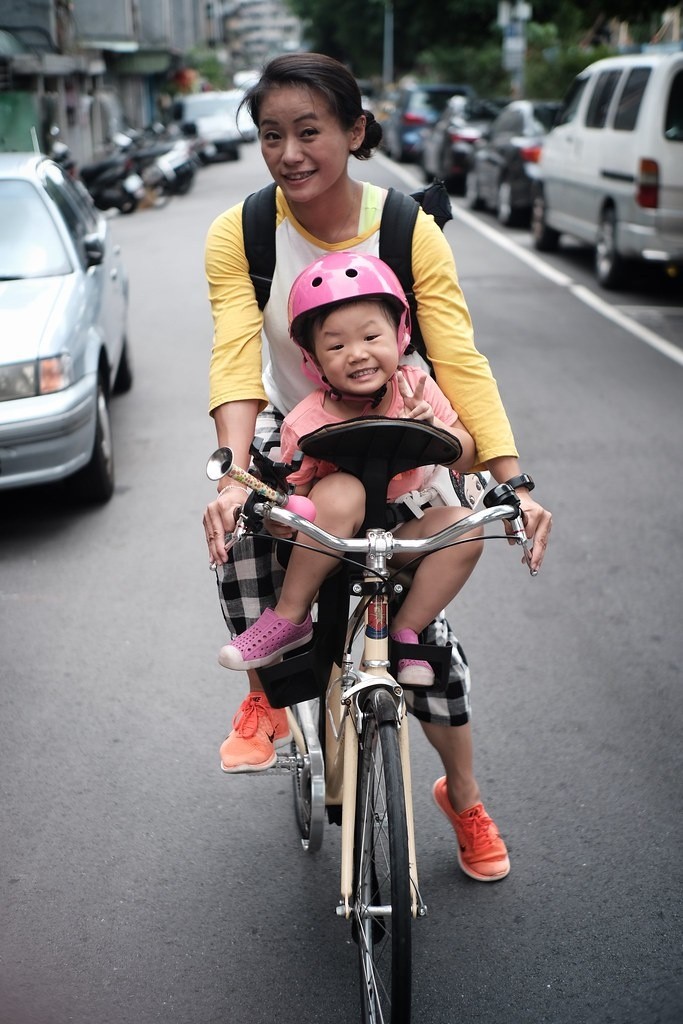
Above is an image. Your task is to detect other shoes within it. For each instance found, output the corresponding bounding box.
[218,608,314,671]
[389,628,434,686]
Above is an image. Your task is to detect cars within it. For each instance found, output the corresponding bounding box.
[0,151,135,505]
[465,98,562,225]
[421,94,511,193]
[356,79,476,160]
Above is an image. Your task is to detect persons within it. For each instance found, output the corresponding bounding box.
[203,55,552,880]
[218,252,484,686]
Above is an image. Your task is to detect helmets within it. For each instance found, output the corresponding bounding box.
[287,252,409,337]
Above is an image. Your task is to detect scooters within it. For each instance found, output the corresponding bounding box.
[47,120,208,215]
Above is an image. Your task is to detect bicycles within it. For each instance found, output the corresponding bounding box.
[208,490,538,1023]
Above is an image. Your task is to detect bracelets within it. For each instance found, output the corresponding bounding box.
[218,485,250,498]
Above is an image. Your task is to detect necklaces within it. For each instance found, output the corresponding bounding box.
[287,191,355,244]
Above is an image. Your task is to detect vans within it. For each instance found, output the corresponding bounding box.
[167,90,258,153]
[531,52,682,292]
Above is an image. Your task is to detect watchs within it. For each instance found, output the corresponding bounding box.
[505,474,535,492]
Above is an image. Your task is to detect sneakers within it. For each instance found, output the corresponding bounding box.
[219,692,292,773]
[432,775,510,882]
